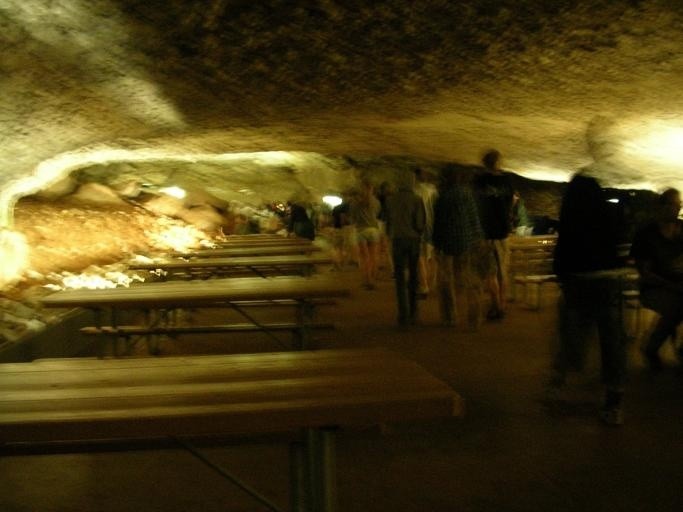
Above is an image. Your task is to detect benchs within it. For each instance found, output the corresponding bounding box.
[509,235,561,312]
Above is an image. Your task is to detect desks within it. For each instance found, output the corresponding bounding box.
[0,254,470,511]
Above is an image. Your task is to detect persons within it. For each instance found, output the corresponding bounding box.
[433,165,488,326]
[383,169,427,326]
[512,188,528,237]
[275,181,392,289]
[628,186,682,368]
[545,173,627,425]
[413,168,440,300]
[471,148,517,319]
